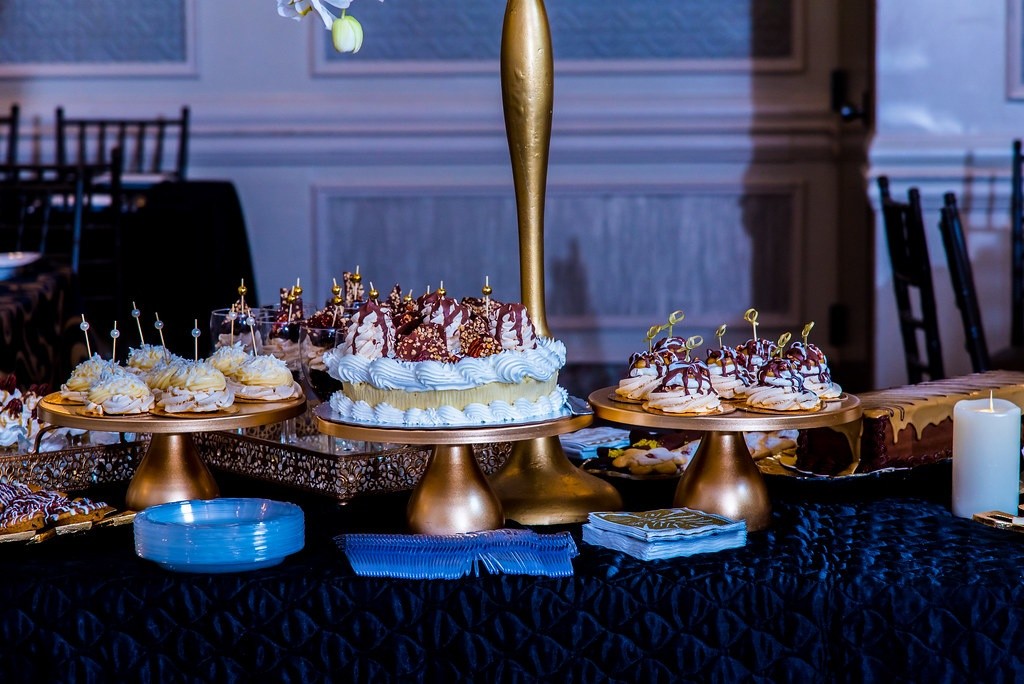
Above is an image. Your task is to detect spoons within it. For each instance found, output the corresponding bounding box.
[332,522,579,582]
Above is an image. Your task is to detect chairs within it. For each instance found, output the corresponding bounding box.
[52,104,193,181]
[0,142,143,362]
[873,137,1023,385]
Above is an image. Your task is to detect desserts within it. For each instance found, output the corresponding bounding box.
[0,273,1024,542]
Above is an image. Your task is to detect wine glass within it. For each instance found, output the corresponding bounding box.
[211,297,391,456]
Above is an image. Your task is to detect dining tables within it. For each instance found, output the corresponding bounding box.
[0,356,1023,682]
[0,173,263,396]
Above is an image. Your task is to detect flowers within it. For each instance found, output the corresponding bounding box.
[277,0,364,56]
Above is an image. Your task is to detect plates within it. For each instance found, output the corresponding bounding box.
[130,495,306,577]
